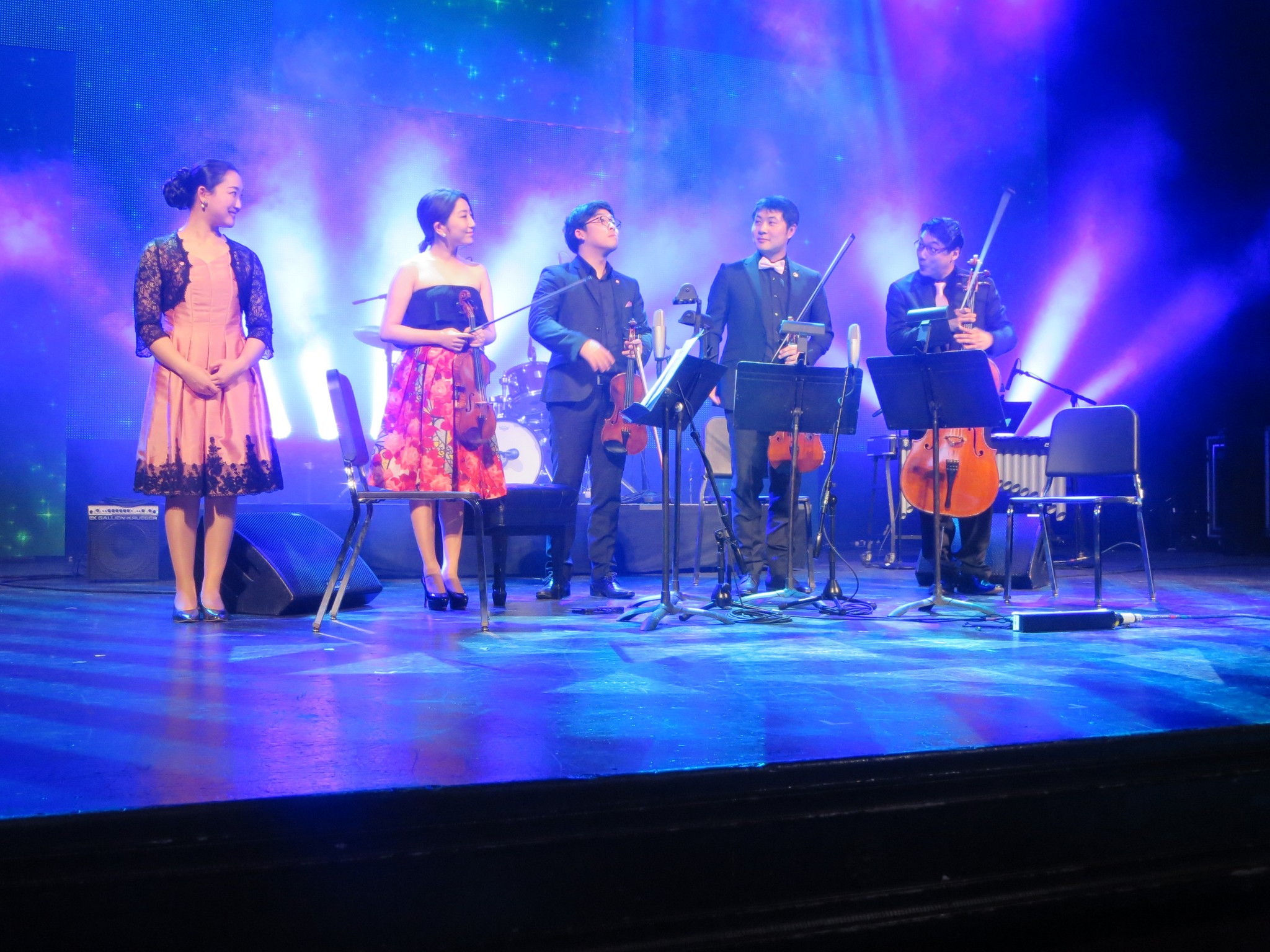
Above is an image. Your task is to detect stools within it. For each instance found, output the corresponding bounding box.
[431,479,568,606]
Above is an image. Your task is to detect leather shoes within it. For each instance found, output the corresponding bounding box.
[928,580,955,595]
[589,571,635,598]
[957,574,1004,594]
[536,571,570,599]
[765,578,813,594]
[736,573,758,595]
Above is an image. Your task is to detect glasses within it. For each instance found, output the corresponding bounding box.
[914,240,945,255]
[585,216,621,229]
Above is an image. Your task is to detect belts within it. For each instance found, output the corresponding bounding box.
[595,374,609,385]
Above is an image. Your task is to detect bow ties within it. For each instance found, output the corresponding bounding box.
[758,256,785,275]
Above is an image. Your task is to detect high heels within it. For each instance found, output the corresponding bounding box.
[444,583,469,609]
[421,576,449,611]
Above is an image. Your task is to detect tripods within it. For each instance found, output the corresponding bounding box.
[617,348,1015,631]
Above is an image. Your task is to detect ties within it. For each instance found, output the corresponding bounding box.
[934,283,948,307]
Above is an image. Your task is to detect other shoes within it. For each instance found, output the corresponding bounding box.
[172,603,200,623]
[199,590,229,622]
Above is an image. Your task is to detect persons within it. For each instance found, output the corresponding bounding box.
[885,217,1019,596]
[701,195,835,597]
[131,158,286,622]
[364,187,507,611]
[527,200,654,598]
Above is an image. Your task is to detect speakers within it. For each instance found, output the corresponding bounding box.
[914,514,1054,589]
[194,513,384,617]
[89,505,161,582]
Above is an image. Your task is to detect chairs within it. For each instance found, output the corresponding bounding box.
[693,416,815,592]
[311,368,489,634]
[1003,405,1160,607]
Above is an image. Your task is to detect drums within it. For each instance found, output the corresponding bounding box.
[494,418,544,484]
[505,361,549,415]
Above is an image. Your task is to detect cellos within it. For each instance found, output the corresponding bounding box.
[899,254,1002,557]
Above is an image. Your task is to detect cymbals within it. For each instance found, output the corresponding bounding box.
[489,359,497,372]
[353,324,403,352]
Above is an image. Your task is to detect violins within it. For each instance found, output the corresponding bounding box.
[601,318,648,456]
[767,317,826,475]
[453,289,497,448]
